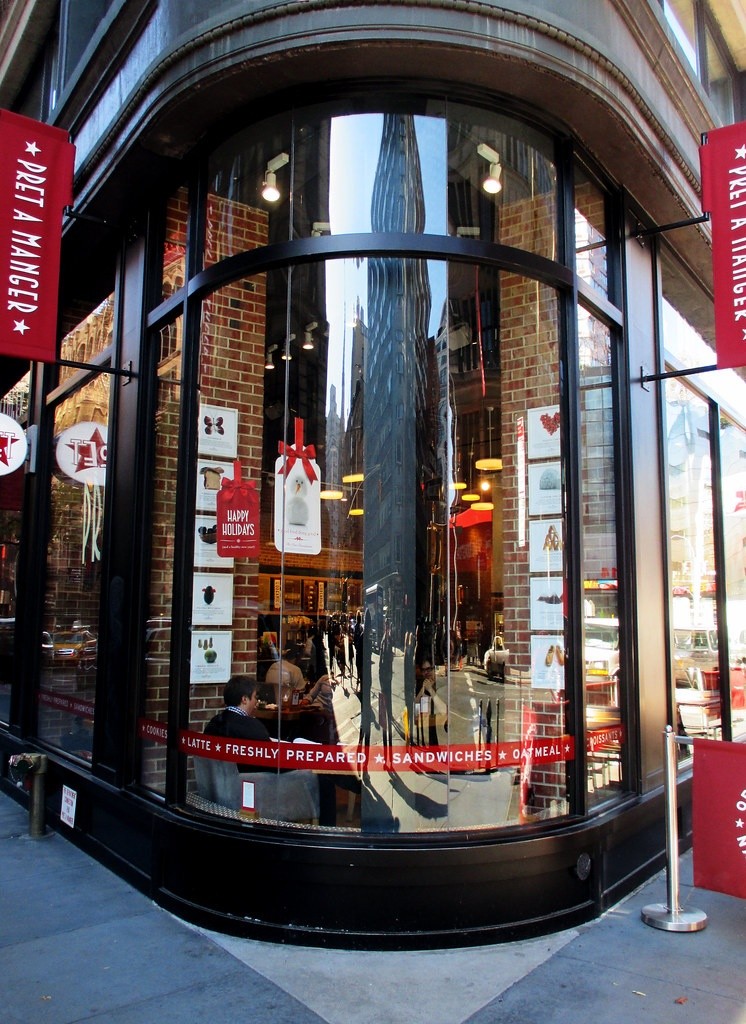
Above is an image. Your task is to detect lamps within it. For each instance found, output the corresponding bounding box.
[320,400,503,517]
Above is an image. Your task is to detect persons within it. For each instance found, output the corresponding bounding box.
[204,673,299,775]
[312,606,503,771]
[299,657,358,745]
[266,645,307,701]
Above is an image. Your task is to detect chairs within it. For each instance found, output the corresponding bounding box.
[194,753,319,825]
